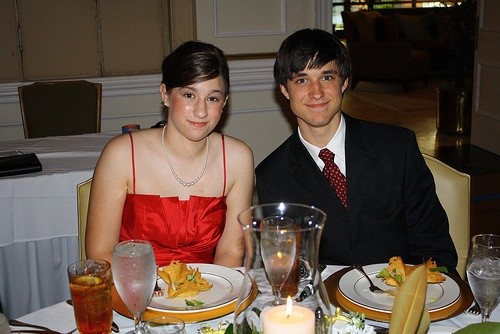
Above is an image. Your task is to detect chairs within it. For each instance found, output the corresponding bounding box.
[422,152,471,280]
[18,79,102,138]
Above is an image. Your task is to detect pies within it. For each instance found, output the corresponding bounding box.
[157,259,213,299]
[376,255,446,292]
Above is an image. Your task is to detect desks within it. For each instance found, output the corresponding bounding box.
[0,131,130,320]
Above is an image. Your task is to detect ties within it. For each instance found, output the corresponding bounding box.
[318,148,347,206]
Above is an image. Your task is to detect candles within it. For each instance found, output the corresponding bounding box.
[261,294,316,334]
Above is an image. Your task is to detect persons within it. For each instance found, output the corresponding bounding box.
[86,40,255,284]
[251,29,467,290]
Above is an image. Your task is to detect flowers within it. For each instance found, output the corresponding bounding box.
[201,306,376,334]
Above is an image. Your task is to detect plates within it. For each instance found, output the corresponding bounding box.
[337,262,462,314]
[145,263,252,314]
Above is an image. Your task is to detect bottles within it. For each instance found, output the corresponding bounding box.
[232,203,332,334]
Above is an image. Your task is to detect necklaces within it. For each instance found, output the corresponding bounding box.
[162,124,209,186]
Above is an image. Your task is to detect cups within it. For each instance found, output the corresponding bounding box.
[144,316,186,334]
[67,259,114,334]
[122,124,140,134]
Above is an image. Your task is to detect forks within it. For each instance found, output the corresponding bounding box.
[467,303,481,315]
[353,263,386,295]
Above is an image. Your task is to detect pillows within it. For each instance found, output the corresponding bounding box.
[356,8,441,43]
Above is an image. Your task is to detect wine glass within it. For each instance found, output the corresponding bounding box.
[466,234,500,322]
[259,216,296,308]
[111,239,157,334]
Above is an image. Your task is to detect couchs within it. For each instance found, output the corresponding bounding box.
[343,9,464,91]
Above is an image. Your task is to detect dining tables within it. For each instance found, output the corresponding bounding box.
[7,266,500,334]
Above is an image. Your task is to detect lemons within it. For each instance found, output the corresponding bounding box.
[71,275,102,291]
